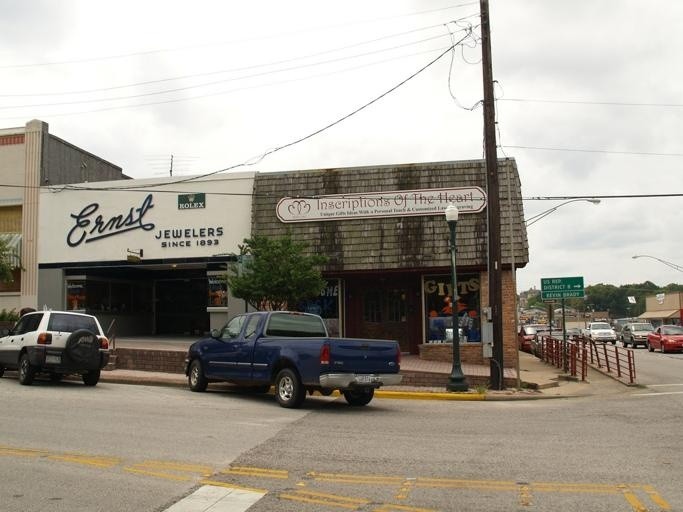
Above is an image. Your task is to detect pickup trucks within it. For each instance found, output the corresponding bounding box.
[178,309,406,409]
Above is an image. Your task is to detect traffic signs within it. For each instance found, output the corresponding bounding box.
[540,276,586,300]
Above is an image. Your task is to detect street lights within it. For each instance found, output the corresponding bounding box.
[522,198,605,226]
[631,254,683,278]
[438,204,471,392]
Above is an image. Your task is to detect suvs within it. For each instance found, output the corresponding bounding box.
[0,304,111,392]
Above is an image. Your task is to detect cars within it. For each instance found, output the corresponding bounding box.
[516,321,683,358]
